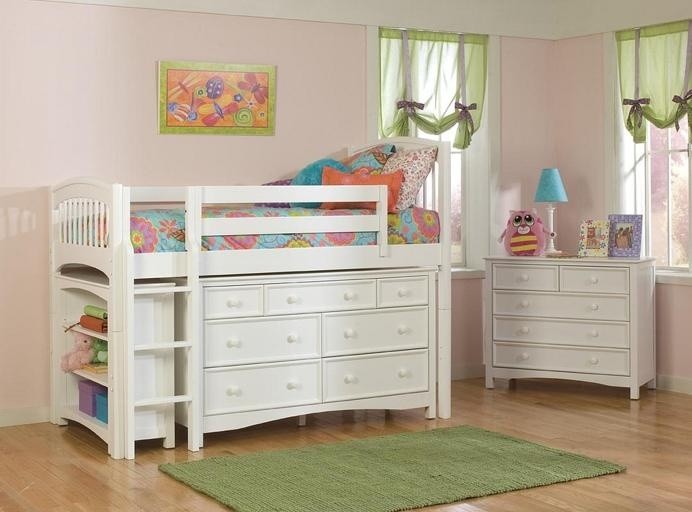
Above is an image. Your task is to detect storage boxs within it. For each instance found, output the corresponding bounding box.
[77,379,109,424]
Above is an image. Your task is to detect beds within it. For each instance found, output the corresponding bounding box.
[51,135,450,276]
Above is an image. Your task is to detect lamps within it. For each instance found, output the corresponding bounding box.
[535,167,567,257]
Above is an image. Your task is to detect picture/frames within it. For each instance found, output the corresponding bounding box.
[578,216,640,256]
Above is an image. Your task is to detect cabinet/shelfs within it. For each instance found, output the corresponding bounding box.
[177,267,451,452]
[47,271,175,460]
[484,253,656,399]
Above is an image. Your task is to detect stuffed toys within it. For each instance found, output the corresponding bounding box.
[61,333,95,373]
[497,208,557,256]
[89,338,108,365]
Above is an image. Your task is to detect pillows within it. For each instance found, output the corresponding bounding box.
[249,142,437,212]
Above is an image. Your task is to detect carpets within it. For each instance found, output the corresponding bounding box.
[160,423,627,511]
